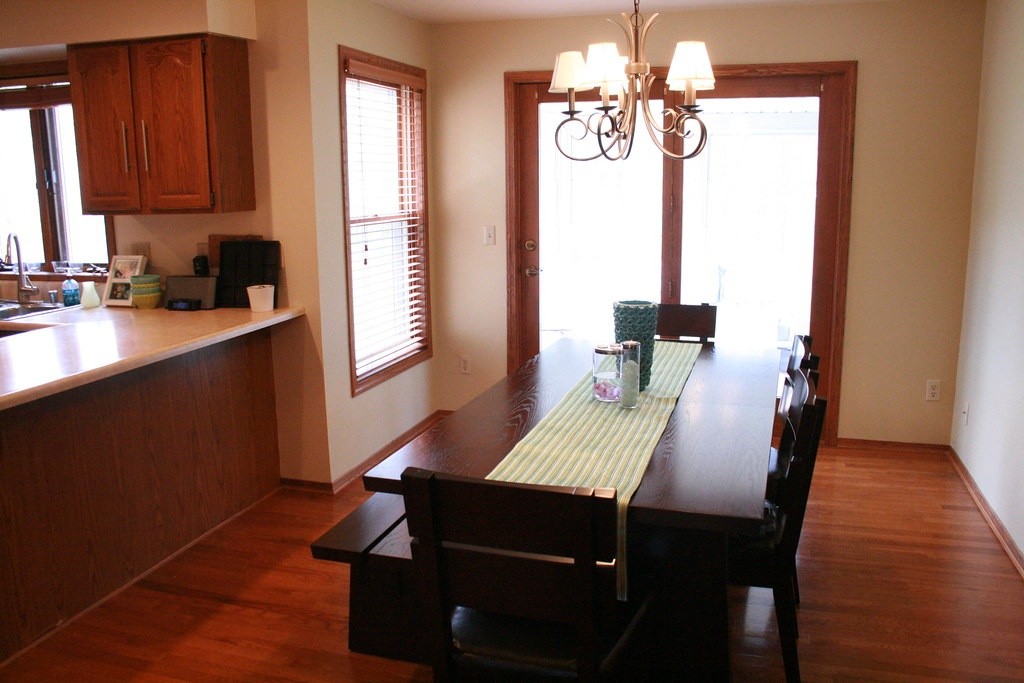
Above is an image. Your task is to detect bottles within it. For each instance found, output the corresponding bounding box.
[80,281,100,309]
[62,275,80,307]
[620,340,640,409]
[593,344,621,403]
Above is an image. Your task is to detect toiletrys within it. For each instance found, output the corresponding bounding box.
[61,267,80,306]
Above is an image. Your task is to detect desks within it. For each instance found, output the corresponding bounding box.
[364,337,782,683]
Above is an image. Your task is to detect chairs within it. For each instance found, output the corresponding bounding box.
[400,306,826,683]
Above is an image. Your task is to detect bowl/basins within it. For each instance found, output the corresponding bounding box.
[130,274,162,310]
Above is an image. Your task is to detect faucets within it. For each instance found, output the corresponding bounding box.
[5,233,39,303]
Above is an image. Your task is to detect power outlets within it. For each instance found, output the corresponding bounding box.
[926,380,941,401]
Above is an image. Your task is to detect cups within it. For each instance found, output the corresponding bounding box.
[51,260,83,274]
[247,285,275,312]
[12,257,41,272]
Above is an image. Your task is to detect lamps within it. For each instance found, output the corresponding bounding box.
[549,0,717,161]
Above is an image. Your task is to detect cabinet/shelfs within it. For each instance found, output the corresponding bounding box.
[67,33,257,216]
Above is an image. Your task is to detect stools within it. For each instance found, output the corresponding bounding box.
[310,490,452,663]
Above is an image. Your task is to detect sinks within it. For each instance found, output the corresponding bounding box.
[0,301,83,322]
